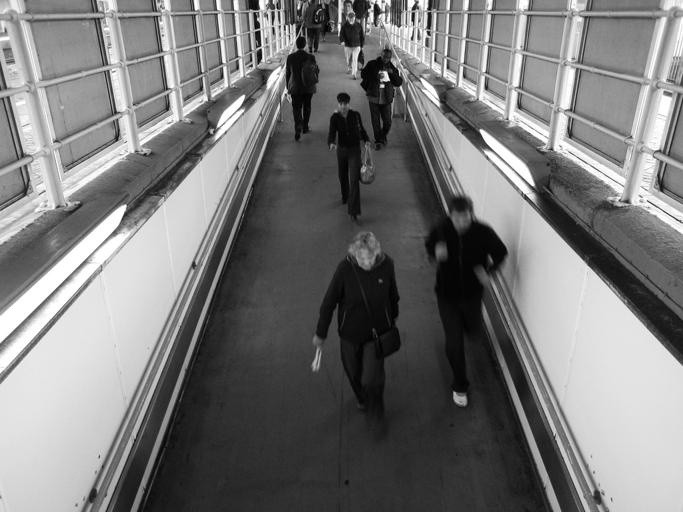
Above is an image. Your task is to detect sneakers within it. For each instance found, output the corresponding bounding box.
[357,398,369,411]
[375,135,388,151]
[453,390,468,408]
[348,68,357,81]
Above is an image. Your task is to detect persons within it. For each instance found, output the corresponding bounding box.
[286,37,316,141]
[374,0,382,27]
[361,48,403,151]
[312,232,400,436]
[384,2,390,24]
[410,0,422,41]
[339,12,365,80]
[327,93,372,222]
[425,196,509,408]
[263,0,372,54]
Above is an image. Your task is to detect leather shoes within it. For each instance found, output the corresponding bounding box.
[351,214,357,224]
[295,127,311,140]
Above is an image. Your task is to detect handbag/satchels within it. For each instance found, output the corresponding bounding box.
[374,327,401,359]
[312,4,325,24]
[360,159,376,184]
[358,51,365,66]
[301,63,319,88]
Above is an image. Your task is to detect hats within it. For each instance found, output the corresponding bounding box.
[337,93,351,102]
